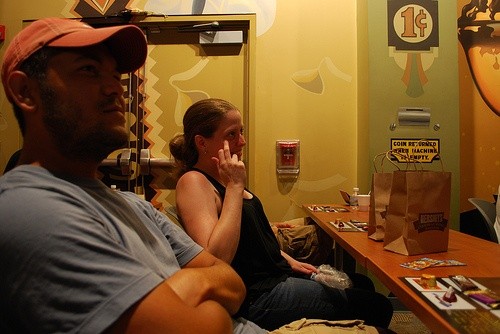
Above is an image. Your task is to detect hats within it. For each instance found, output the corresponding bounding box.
[0,17,148,102]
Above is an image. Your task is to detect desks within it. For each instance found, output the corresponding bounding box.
[302,205,500,334]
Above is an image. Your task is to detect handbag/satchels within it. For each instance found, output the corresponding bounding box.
[367,139,453,256]
[277,224,334,266]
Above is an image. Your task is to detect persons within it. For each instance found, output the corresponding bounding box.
[169,98,394,329]
[0,18,396,334]
